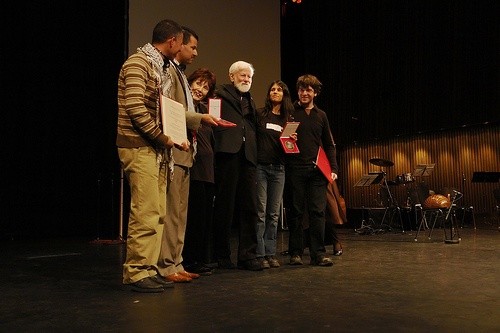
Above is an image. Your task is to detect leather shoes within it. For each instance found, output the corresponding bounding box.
[125,277,165,293]
[150,275,175,287]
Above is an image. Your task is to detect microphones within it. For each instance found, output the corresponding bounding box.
[452,189,462,194]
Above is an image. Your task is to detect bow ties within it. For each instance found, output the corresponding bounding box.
[178,64,187,71]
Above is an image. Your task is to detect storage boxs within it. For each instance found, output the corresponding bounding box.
[280,122,300,154]
[208,97,237,127]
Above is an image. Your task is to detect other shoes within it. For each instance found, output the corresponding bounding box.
[187,265,211,275]
[333,245,343,256]
[310,256,333,266]
[268,258,280,267]
[178,271,200,278]
[238,258,269,270]
[164,272,192,282]
[290,255,303,265]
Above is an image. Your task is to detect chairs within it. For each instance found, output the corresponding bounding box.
[414,189,463,241]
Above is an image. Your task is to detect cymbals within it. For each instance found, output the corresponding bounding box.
[369,159,394,167]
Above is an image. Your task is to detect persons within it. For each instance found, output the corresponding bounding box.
[156,25,221,283]
[116,19,191,292]
[214,60,264,270]
[287,74,337,266]
[186,66,216,274]
[280,181,343,256]
[255,80,298,268]
